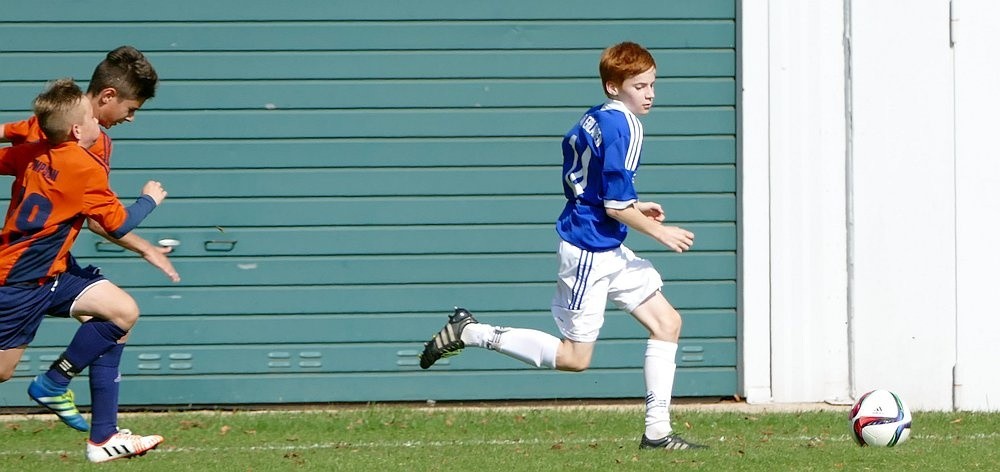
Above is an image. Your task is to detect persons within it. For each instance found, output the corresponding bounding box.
[417,42,712,452]
[0,77,165,432]
[0,45,182,464]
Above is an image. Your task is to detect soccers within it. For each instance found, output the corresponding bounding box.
[849,388,913,447]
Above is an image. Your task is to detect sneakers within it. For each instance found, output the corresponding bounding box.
[86,426,164,463]
[27,374,89,432]
[639,430,710,452]
[419,307,478,369]
